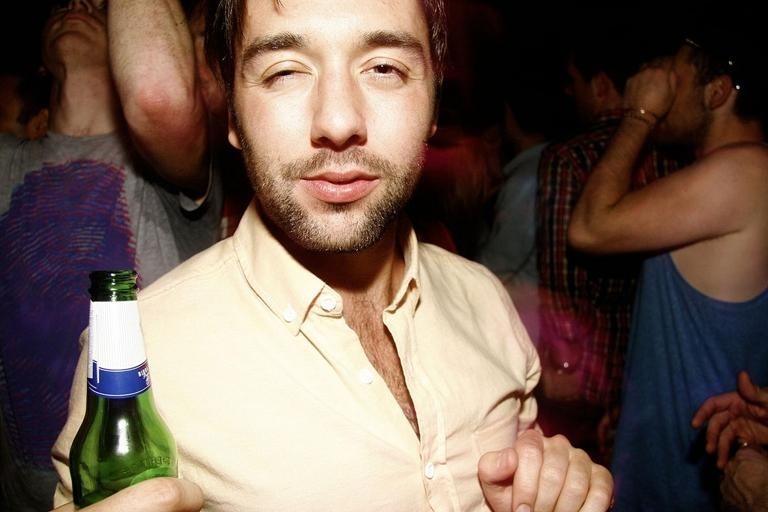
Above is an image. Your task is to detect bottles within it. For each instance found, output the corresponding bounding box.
[68,268,186,507]
